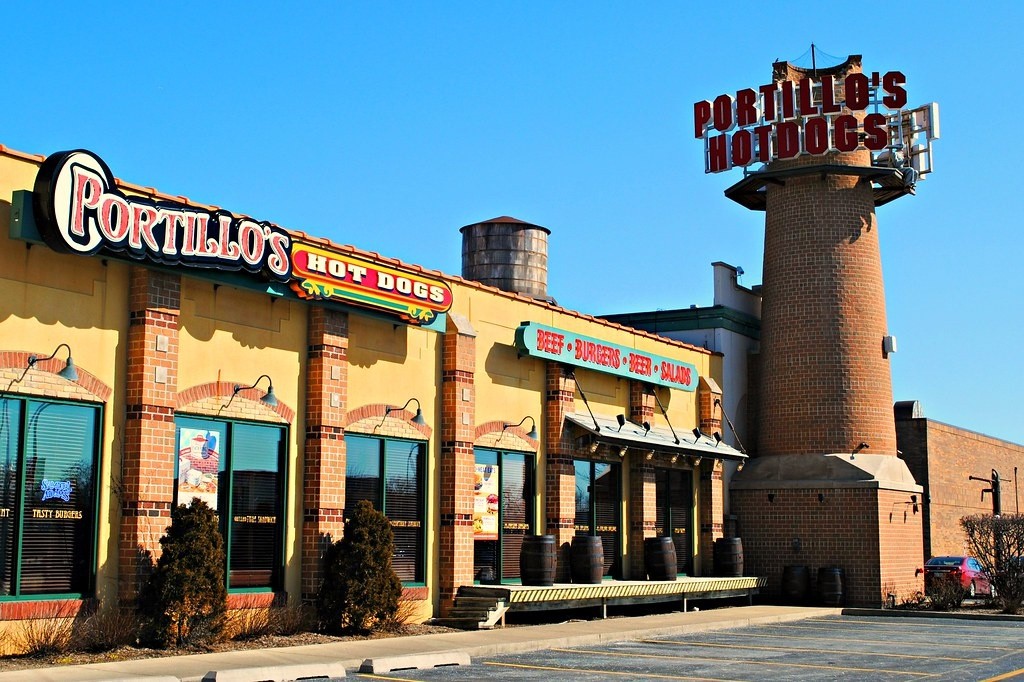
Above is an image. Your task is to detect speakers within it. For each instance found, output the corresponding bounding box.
[885,335,896,353]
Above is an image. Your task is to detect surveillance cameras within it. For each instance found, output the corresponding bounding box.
[737,266,744,274]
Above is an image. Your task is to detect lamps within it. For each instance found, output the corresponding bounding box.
[713,431,722,447]
[737,457,745,472]
[645,448,656,460]
[897,449,902,455]
[906,495,919,505]
[234,374,279,407]
[670,451,679,464]
[386,397,425,425]
[28,344,79,381]
[643,421,650,437]
[589,436,601,453]
[716,457,724,468]
[768,493,776,503]
[503,415,539,440]
[618,444,628,458]
[818,493,825,503]
[860,441,869,449]
[616,414,625,433]
[692,427,701,444]
[693,454,703,466]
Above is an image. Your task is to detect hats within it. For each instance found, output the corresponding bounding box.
[894,139,904,145]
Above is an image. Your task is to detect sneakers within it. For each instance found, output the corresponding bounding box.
[904,186,916,196]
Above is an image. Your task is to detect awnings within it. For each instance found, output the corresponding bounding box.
[564,413,750,462]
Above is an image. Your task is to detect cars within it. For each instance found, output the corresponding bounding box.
[925,556,998,599]
[988,556,1024,592]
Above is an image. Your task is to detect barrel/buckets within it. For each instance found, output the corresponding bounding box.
[817,567,845,607]
[644,536,678,580]
[715,536,744,577]
[782,567,811,605]
[571,535,604,584]
[520,534,557,587]
